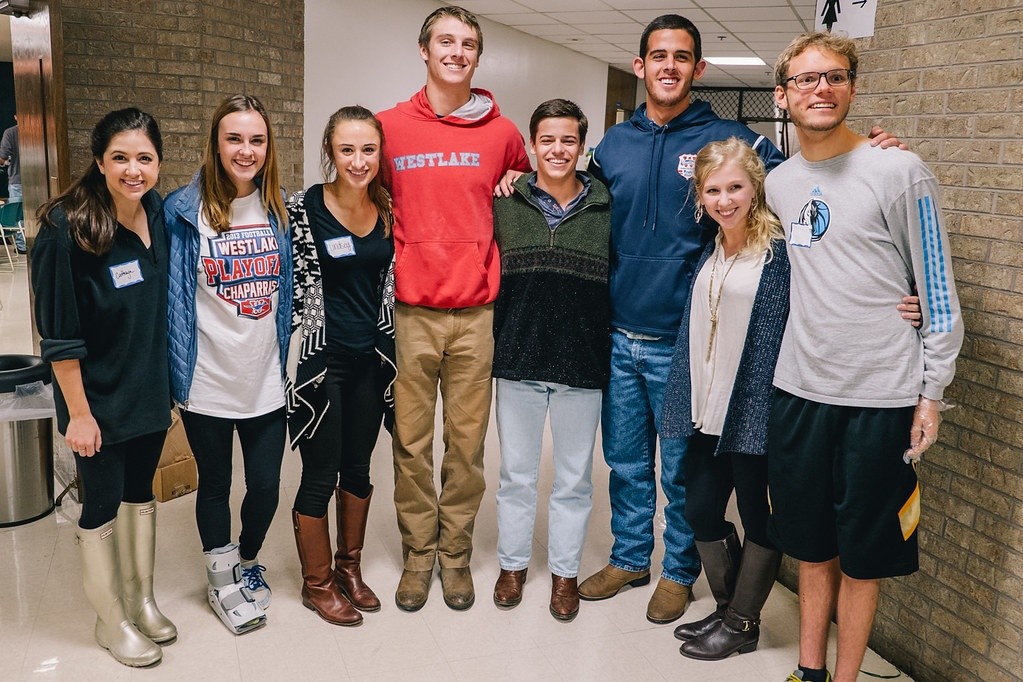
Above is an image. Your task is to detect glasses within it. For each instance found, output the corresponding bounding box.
[786,69,854,90]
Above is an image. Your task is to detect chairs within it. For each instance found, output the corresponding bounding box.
[0,201,26,273]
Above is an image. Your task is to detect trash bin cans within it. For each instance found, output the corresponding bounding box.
[0,354,55,527]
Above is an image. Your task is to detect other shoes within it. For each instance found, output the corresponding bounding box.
[784,669,832,682]
[646,577,692,624]
[577,565,650,601]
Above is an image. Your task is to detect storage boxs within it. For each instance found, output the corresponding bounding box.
[156,404,195,469]
[153,456,198,503]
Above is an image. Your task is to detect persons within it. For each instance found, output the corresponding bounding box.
[164,93,293,637]
[654,137,921,663]
[491,97,613,622]
[754,32,966,682]
[0,111,27,254]
[371,4,534,613]
[29,106,180,669]
[284,105,399,627]
[493,13,910,624]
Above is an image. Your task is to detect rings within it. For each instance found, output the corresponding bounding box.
[78,449,85,451]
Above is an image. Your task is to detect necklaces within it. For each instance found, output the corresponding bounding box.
[704,223,743,365]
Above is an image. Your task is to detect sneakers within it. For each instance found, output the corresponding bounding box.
[241,558,271,609]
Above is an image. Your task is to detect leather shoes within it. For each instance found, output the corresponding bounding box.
[494,567,528,605]
[395,569,432,611]
[550,573,579,620]
[440,567,475,610]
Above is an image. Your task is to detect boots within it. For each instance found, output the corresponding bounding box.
[674,521,742,641]
[292,507,363,626]
[116,495,178,642]
[680,536,782,661]
[76,518,163,667]
[334,485,381,612]
